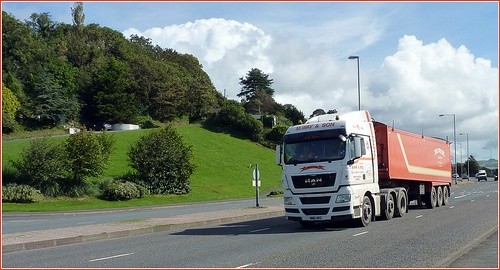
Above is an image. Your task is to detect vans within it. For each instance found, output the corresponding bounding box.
[477,170,488,181]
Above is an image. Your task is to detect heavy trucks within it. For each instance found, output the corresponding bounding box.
[277,110,452,227]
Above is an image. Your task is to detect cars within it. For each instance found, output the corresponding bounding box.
[475,173,478,178]
[452,174,459,178]
[462,174,469,179]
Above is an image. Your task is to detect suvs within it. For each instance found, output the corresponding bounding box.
[493,169,498,181]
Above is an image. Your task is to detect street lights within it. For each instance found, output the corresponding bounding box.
[482,147,492,159]
[348,56,360,111]
[438,114,458,184]
[451,142,462,163]
[459,133,469,180]
